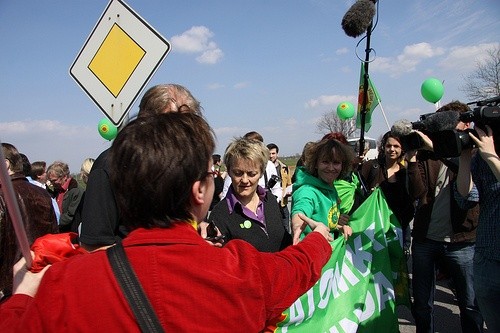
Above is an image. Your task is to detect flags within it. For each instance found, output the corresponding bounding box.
[355,62,382,132]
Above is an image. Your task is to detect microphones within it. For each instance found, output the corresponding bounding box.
[423,111,473,130]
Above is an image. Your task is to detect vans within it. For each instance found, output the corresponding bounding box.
[346,137,380,162]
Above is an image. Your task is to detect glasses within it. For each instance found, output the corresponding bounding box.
[208,165,221,179]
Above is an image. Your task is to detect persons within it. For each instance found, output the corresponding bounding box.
[356,102,500,333]
[291,138,355,245]
[220,131,282,203]
[80,84,223,249]
[0,112,331,333]
[324,132,364,223]
[267,143,291,207]
[0,144,100,300]
[209,137,289,252]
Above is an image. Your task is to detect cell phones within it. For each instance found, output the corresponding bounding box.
[206,235,225,240]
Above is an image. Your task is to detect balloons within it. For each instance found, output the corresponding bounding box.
[337,102,354,120]
[98,117,117,142]
[421,78,445,104]
